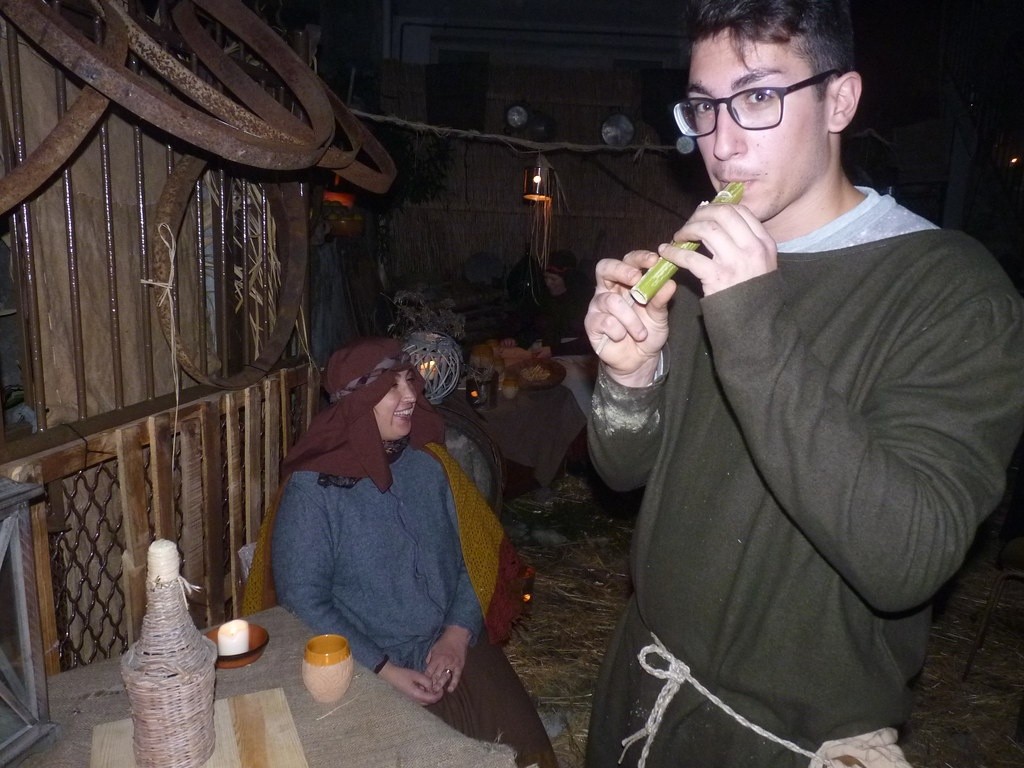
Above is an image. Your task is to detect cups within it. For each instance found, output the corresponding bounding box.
[495,359,504,390]
[467,369,498,412]
[302,634,351,702]
[471,346,494,368]
[503,379,519,399]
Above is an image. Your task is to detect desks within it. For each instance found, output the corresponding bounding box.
[0,607,519,768]
[448,354,591,489]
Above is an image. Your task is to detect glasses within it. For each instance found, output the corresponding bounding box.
[674,69,842,138]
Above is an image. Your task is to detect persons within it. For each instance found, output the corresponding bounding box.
[493,251,594,354]
[241,339,560,768]
[581,0,1024,768]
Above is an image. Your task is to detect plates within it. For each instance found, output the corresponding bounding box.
[510,359,566,392]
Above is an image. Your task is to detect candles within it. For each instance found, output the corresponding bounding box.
[218,619,249,656]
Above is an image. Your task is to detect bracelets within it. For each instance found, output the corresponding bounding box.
[375,654,389,673]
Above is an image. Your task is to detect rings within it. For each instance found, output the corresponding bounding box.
[443,669,452,673]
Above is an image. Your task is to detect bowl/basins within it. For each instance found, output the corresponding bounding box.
[203,623,270,668]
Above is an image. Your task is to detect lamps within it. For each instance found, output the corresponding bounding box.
[522,151,551,202]
[402,331,460,407]
[465,367,499,411]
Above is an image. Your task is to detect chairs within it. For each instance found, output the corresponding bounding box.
[239,442,528,646]
[961,537,1024,683]
[439,404,507,520]
